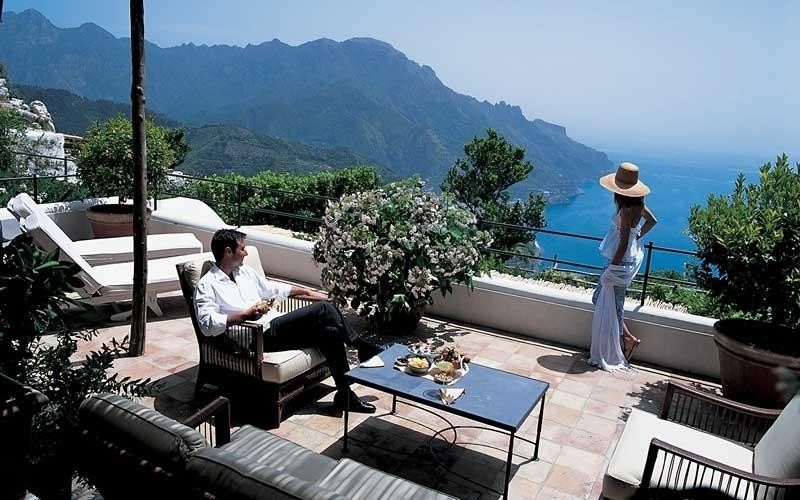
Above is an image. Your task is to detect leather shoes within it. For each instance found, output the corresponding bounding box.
[357,341,396,364]
[332,389,377,414]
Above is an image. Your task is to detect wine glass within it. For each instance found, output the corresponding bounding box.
[436,361,454,385]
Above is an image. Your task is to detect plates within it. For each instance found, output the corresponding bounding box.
[408,342,436,357]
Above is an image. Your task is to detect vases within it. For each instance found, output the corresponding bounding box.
[376,300,427,334]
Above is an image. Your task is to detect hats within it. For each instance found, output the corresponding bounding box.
[597,161,651,198]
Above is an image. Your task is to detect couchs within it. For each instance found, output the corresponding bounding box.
[601,375,799,498]
[75,391,459,500]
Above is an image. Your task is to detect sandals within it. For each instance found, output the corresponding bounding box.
[586,358,604,371]
[623,335,642,361]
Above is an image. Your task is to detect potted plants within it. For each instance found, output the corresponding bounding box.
[683,154,800,409]
[74,115,178,238]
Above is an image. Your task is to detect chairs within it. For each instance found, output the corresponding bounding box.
[6,193,203,266]
[17,213,266,322]
[177,245,350,429]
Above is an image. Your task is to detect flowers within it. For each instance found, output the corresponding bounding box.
[312,174,494,318]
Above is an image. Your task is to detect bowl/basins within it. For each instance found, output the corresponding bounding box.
[407,364,430,374]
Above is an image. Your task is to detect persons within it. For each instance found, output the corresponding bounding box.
[584,191,657,373]
[193,230,402,413]
